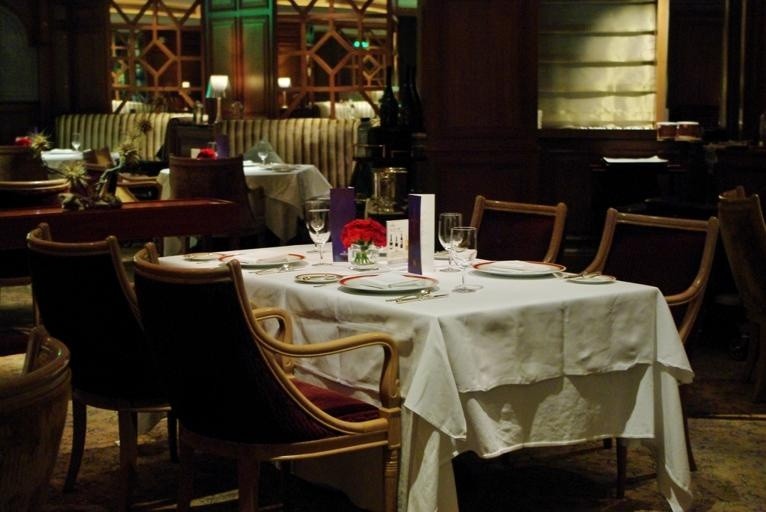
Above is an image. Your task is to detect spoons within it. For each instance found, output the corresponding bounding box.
[386,288,431,301]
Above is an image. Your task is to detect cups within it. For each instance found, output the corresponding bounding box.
[206,140,218,153]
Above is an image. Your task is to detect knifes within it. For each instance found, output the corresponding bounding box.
[392,294,449,304]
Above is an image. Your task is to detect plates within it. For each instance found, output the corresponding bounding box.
[296,272,341,284]
[219,252,304,266]
[567,274,617,286]
[341,273,438,294]
[184,252,225,261]
[470,260,567,278]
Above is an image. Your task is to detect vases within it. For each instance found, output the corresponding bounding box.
[347,244,376,267]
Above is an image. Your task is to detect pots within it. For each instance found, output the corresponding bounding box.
[369,165,413,211]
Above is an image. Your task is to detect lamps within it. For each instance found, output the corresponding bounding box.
[275,76,291,110]
[659,121,705,163]
[207,74,233,124]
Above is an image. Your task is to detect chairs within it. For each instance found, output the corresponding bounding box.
[0,132,169,205]
[169,154,254,230]
[0,328,70,512]
[578,206,719,499]
[719,185,766,348]
[131,243,402,511]
[16,223,179,488]
[462,194,568,265]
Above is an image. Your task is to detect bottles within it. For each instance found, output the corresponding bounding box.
[192,102,203,124]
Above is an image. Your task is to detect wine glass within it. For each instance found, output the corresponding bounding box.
[70,132,81,153]
[306,208,337,267]
[448,225,480,294]
[256,141,269,167]
[302,198,332,253]
[438,210,464,272]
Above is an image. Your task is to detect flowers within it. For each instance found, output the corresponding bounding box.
[193,149,220,159]
[339,219,386,262]
